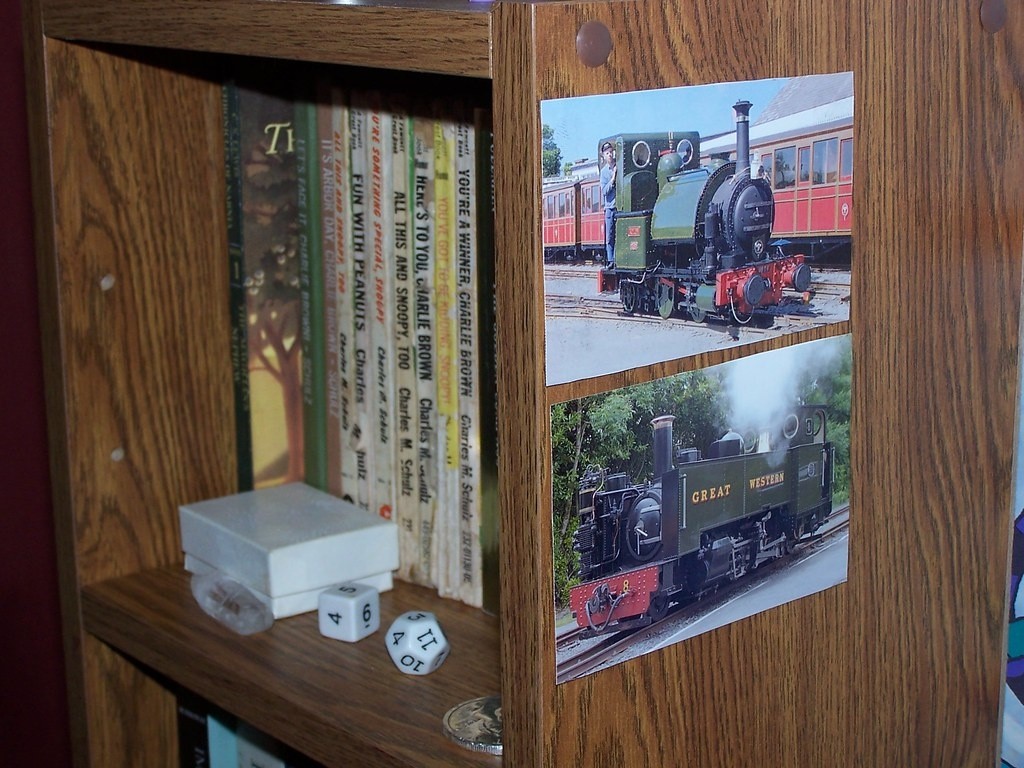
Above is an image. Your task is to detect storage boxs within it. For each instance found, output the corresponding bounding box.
[179,484,401,621]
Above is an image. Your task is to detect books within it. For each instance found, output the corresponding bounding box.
[218,73,499,609]
[175,699,285,768]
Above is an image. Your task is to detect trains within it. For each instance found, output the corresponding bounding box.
[542,115,853,264]
[568,403,835,639]
[598,98,815,327]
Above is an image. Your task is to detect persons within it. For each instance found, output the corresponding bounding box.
[596,142,618,269]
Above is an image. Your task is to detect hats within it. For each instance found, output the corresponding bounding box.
[602,142,611,152]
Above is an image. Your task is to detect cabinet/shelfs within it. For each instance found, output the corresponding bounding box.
[20,0,1024,768]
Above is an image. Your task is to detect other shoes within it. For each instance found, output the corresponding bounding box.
[608,262,614,268]
